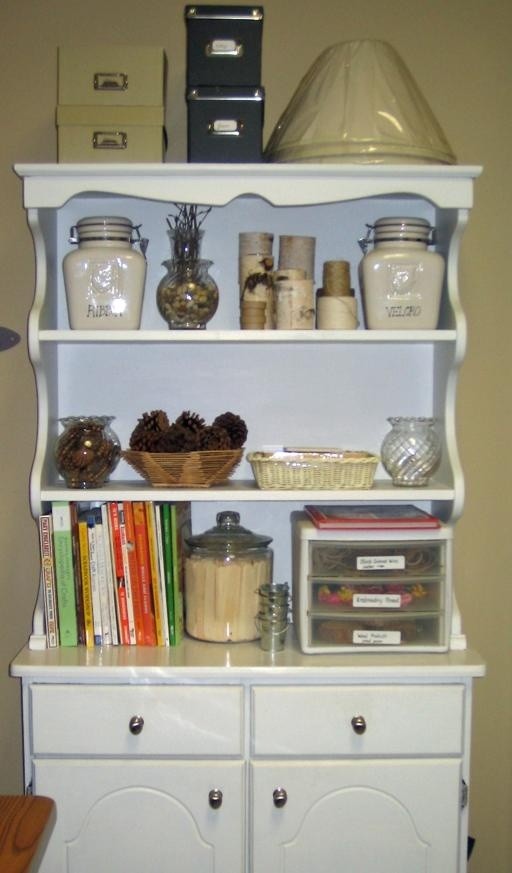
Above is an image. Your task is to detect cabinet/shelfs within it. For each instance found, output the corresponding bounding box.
[11,153,482,653]
[4,621,488,873]
[290,509,455,654]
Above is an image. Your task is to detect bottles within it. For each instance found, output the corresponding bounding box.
[357,216,445,330]
[53,415,121,489]
[380,416,442,487]
[184,509,274,644]
[61,215,147,330]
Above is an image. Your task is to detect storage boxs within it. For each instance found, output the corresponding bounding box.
[183,3,266,165]
[53,40,169,163]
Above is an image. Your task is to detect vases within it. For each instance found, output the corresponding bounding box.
[156,228,219,329]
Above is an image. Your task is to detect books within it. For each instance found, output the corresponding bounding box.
[304,502,443,532]
[38,494,193,650]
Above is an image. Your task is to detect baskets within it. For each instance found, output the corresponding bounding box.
[121,449,245,489]
[246,449,379,492]
